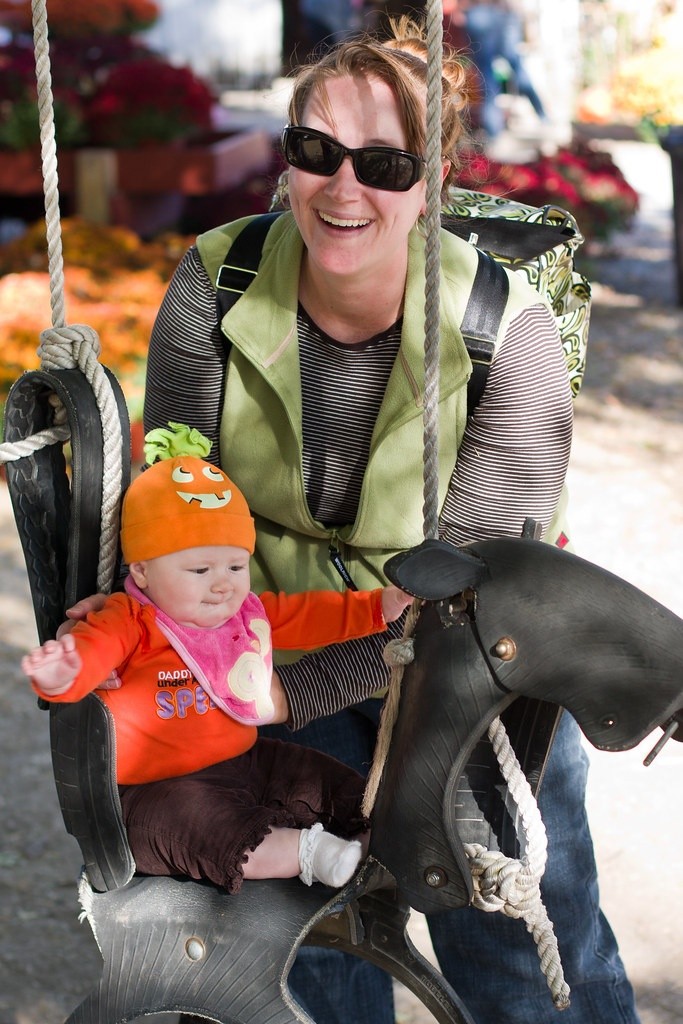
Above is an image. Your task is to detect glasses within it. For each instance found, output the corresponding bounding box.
[281,122,428,192]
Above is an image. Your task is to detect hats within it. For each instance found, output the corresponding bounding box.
[119,421,256,565]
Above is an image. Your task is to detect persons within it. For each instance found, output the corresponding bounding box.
[19,422,427,894]
[56,15,642,1024]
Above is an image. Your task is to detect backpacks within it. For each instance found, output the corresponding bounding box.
[214,167,591,401]
